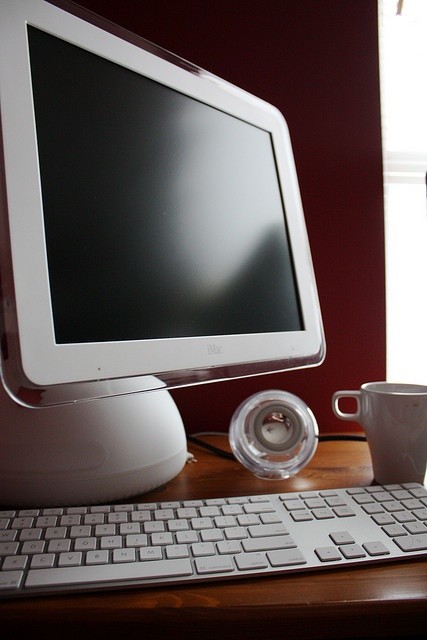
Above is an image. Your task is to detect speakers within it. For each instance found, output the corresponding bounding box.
[228,388,321,481]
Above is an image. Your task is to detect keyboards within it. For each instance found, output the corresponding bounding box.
[0,481,427,600]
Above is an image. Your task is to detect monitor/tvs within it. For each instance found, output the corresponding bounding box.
[0,0,328,508]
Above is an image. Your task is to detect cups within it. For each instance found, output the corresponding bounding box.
[332,381,427,485]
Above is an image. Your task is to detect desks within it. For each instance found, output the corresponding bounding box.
[1,432,426,640]
